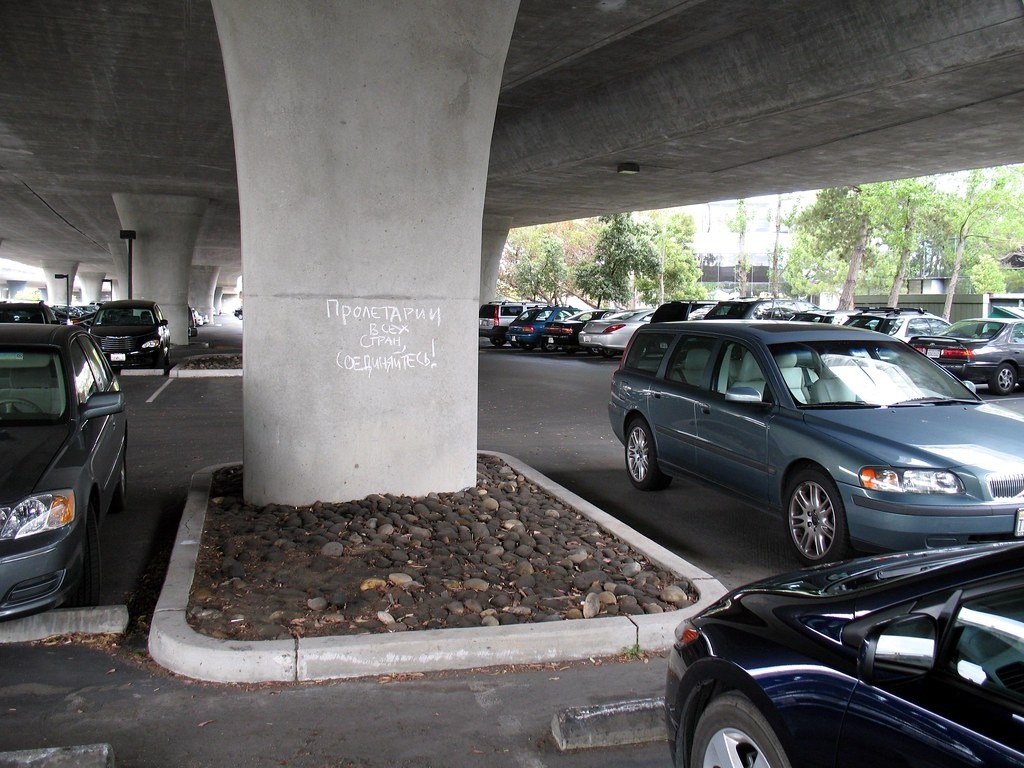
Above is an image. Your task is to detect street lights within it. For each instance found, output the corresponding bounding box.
[55,274,70,318]
[120,229,137,299]
[101,279,112,302]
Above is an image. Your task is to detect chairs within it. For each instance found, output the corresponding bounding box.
[811,358,859,403]
[765,352,810,406]
[6,368,53,412]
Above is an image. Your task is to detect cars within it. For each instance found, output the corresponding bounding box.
[505,305,589,351]
[907,317,1024,396]
[608,319,1024,567]
[577,308,657,358]
[0,322,127,623]
[0,300,208,339]
[540,309,621,355]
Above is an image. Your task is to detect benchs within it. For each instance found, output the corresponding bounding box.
[670,350,766,394]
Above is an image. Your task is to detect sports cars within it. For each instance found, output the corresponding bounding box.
[663,535,1024,768]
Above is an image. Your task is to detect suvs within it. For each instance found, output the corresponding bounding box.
[653,296,952,361]
[84,299,170,373]
[479,300,573,348]
